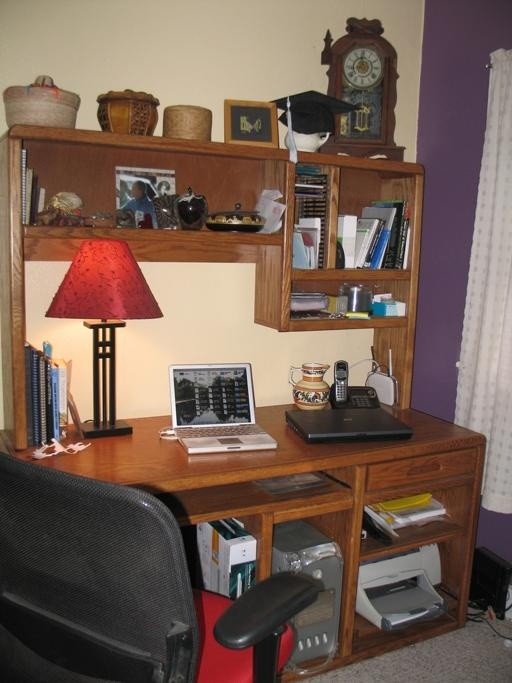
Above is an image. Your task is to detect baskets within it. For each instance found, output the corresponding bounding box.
[4,72,82,132]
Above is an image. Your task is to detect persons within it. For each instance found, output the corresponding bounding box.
[152,181,177,229]
[120,181,158,228]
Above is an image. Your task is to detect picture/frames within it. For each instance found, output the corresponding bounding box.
[221,99,282,151]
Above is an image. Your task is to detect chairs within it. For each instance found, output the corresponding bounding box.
[1,449,319,683]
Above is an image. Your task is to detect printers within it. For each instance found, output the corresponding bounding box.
[355,543,448,632]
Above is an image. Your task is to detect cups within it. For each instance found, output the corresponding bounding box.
[342,286,369,313]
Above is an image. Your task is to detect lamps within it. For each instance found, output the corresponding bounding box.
[45,241,169,439]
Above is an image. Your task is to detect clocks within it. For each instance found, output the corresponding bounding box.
[320,15,406,161]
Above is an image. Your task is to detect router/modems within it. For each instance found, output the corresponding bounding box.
[367,345,399,406]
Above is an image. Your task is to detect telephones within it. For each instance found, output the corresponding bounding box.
[329,360,380,409]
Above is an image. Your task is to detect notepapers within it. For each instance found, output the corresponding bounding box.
[346,312,371,320]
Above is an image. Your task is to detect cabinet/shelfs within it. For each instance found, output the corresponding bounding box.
[1,125,489,683]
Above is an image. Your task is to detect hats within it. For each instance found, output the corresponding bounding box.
[268,89,359,133]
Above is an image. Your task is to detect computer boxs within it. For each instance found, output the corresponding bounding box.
[271,521,343,669]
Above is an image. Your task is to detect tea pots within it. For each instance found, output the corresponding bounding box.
[287,361,332,411]
[284,128,330,153]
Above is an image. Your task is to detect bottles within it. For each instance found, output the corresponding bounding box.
[174,187,208,231]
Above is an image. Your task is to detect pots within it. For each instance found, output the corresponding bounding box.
[204,204,265,233]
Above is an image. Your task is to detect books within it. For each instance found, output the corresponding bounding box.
[369,493,447,531]
[292,165,410,271]
[20,149,46,227]
[24,339,71,448]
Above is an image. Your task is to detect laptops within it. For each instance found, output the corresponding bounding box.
[285,407,413,444]
[170,363,278,454]
